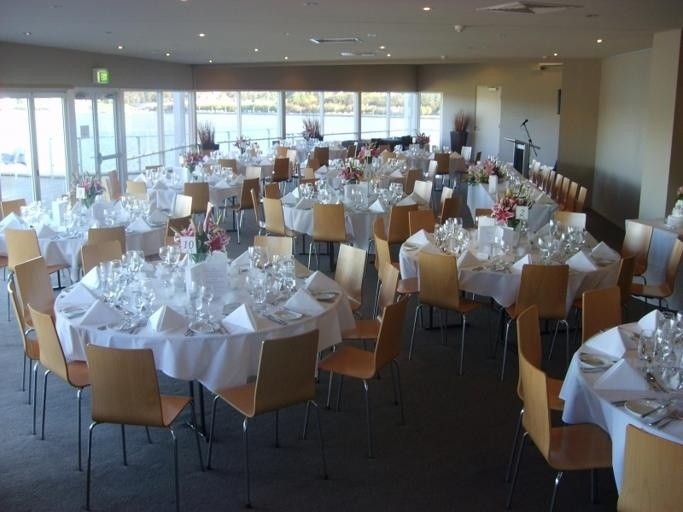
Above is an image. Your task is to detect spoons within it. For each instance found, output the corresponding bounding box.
[647,372,667,394]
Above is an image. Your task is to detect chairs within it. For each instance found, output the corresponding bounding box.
[307,294,412,460]
[503,303,565,486]
[407,251,497,377]
[630,238,683,313]
[611,423,683,512]
[210,328,329,512]
[579,284,624,345]
[25,300,154,472]
[80,342,204,511]
[502,348,613,512]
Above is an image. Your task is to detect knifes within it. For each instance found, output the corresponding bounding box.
[641,402,671,418]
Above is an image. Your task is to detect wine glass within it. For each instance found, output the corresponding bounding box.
[299,144,579,266]
[20,164,233,231]
[100,245,295,319]
[638,311,683,373]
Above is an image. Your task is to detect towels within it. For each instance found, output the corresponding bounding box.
[291,181,416,213]
[593,358,651,393]
[583,326,639,359]
[407,217,620,274]
[64,246,344,337]
[637,308,666,339]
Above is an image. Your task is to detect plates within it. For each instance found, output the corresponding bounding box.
[106,320,136,331]
[315,293,336,299]
[624,399,668,420]
[188,320,220,334]
[578,356,612,369]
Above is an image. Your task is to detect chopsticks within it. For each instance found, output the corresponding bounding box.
[613,399,656,406]
